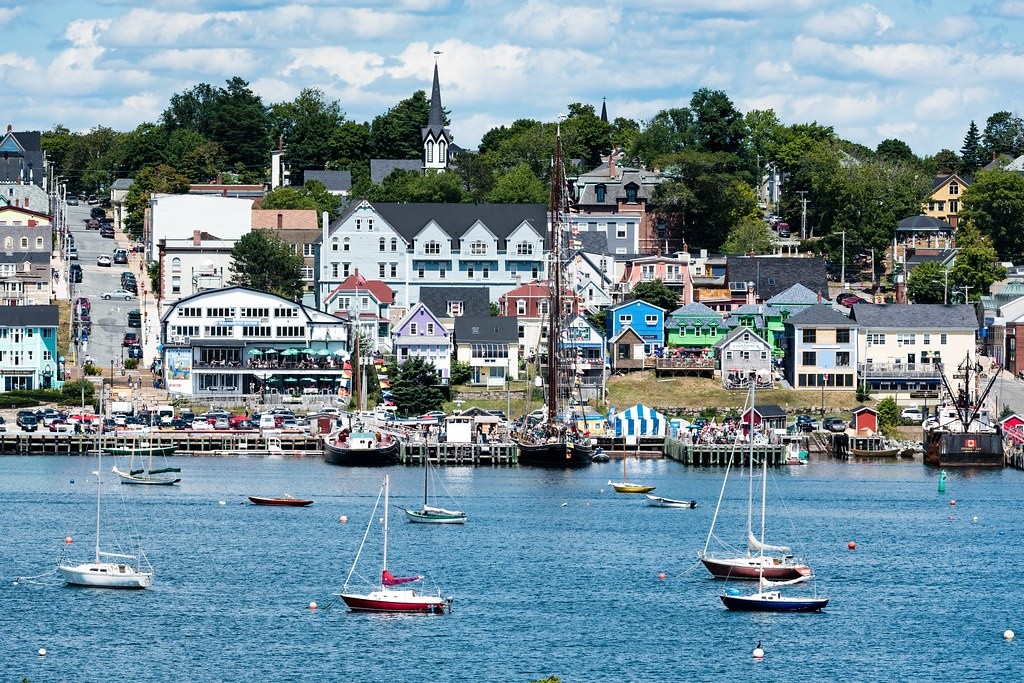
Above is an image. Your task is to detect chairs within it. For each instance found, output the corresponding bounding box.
[195,359,337,369]
[267,388,338,395]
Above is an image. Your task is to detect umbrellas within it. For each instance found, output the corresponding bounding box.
[268,375,342,382]
[668,346,712,354]
[246,348,348,369]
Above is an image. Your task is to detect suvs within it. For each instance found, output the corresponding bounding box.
[901,408,935,424]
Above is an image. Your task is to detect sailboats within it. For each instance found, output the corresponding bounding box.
[59,392,157,589]
[608,426,656,492]
[695,382,829,613]
[111,397,182,484]
[393,433,468,524]
[331,474,446,613]
[511,125,610,468]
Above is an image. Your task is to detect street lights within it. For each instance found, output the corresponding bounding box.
[110,360,114,386]
[121,343,124,365]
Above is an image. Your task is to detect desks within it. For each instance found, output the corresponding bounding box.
[337,362,345,369]
[231,361,240,367]
[287,388,295,395]
[198,361,205,367]
[304,361,310,368]
[319,361,328,370]
[212,361,223,368]
[271,388,279,394]
[285,361,293,368]
[251,362,259,368]
[322,388,329,394]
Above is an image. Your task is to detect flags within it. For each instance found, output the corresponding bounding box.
[372,350,393,405]
[337,362,352,405]
[565,180,586,458]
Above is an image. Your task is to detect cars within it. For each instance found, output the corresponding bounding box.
[62,190,98,206]
[84,207,115,238]
[73,298,92,344]
[796,414,846,433]
[96,248,128,267]
[127,310,144,328]
[123,333,143,358]
[826,260,861,282]
[836,293,868,308]
[14,405,543,431]
[769,212,790,237]
[100,272,138,302]
[694,418,707,428]
[62,225,77,259]
[69,264,83,283]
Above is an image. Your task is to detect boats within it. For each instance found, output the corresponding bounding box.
[324,414,398,465]
[920,349,1007,468]
[101,446,179,455]
[249,496,314,506]
[851,449,901,457]
[646,495,698,508]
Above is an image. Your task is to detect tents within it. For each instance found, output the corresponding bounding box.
[614,403,666,434]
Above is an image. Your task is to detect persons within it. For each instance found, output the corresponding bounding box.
[139,260,143,273]
[211,354,344,369]
[121,363,125,376]
[666,353,707,367]
[128,375,132,388]
[138,375,142,389]
[245,405,248,417]
[385,420,445,437]
[688,421,738,444]
[150,360,163,389]
[254,405,259,414]
[249,381,339,395]
[54,271,59,284]
